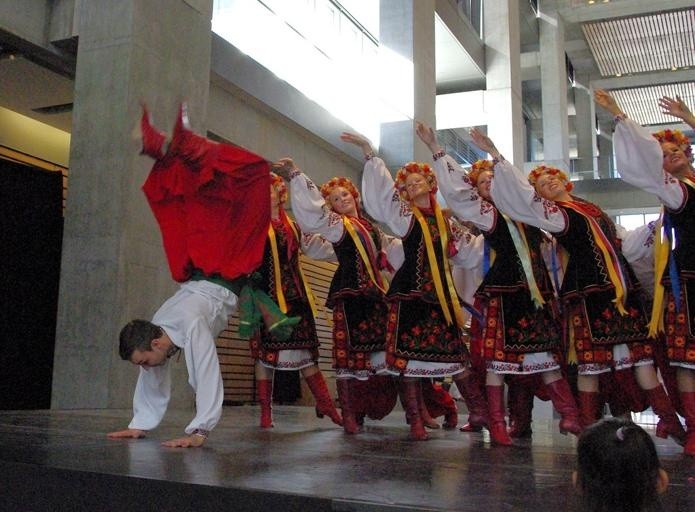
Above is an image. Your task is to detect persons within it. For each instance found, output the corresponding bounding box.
[595,89,695,454]
[415,120,585,438]
[340,133,487,439]
[107,100,302,448]
[469,128,686,446]
[572,419,669,511]
[253,157,437,436]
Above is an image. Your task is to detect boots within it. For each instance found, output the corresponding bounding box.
[336,374,537,450]
[171,98,207,162]
[130,99,165,163]
[306,371,344,426]
[679,389,695,457]
[644,382,685,448]
[256,378,275,427]
[544,377,586,440]
